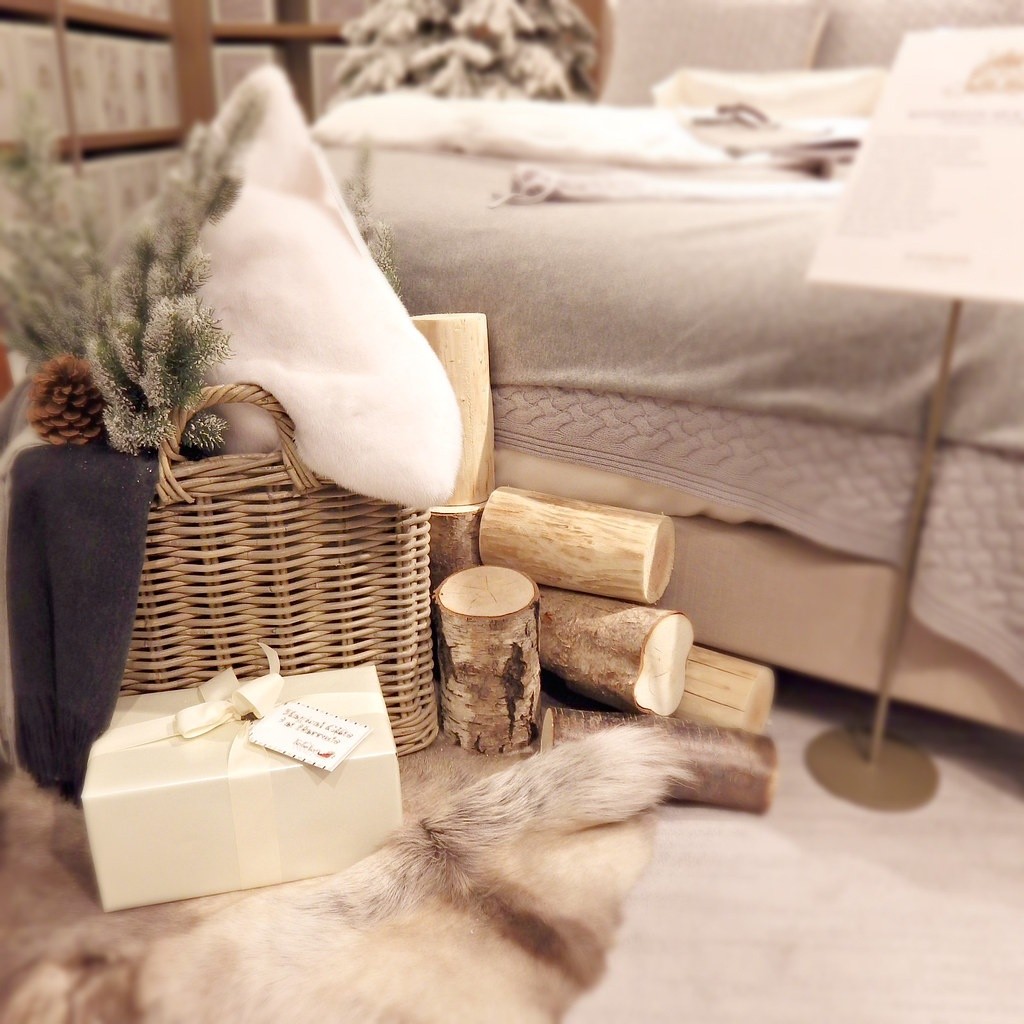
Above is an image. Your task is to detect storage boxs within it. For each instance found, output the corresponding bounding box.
[81,666,402,914]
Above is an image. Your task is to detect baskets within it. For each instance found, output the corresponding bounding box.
[4,382,440,756]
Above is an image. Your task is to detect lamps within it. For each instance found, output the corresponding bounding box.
[805,21,1024,813]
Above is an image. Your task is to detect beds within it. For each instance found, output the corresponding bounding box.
[311,95,1024,740]
